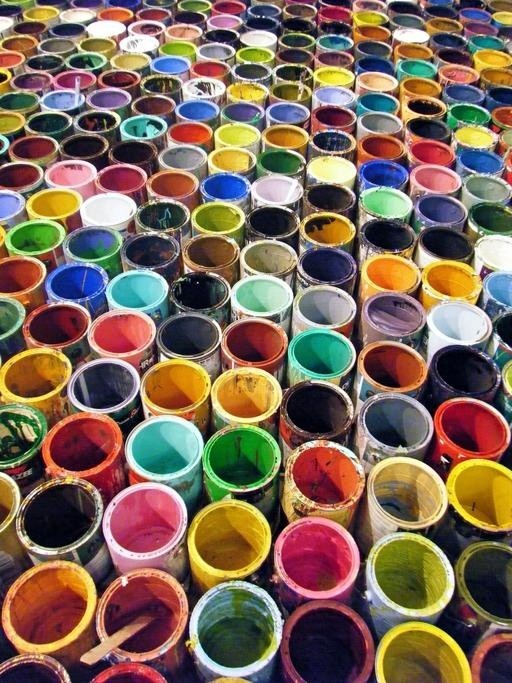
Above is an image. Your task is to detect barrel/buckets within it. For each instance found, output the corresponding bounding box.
[0,0,512,683]
